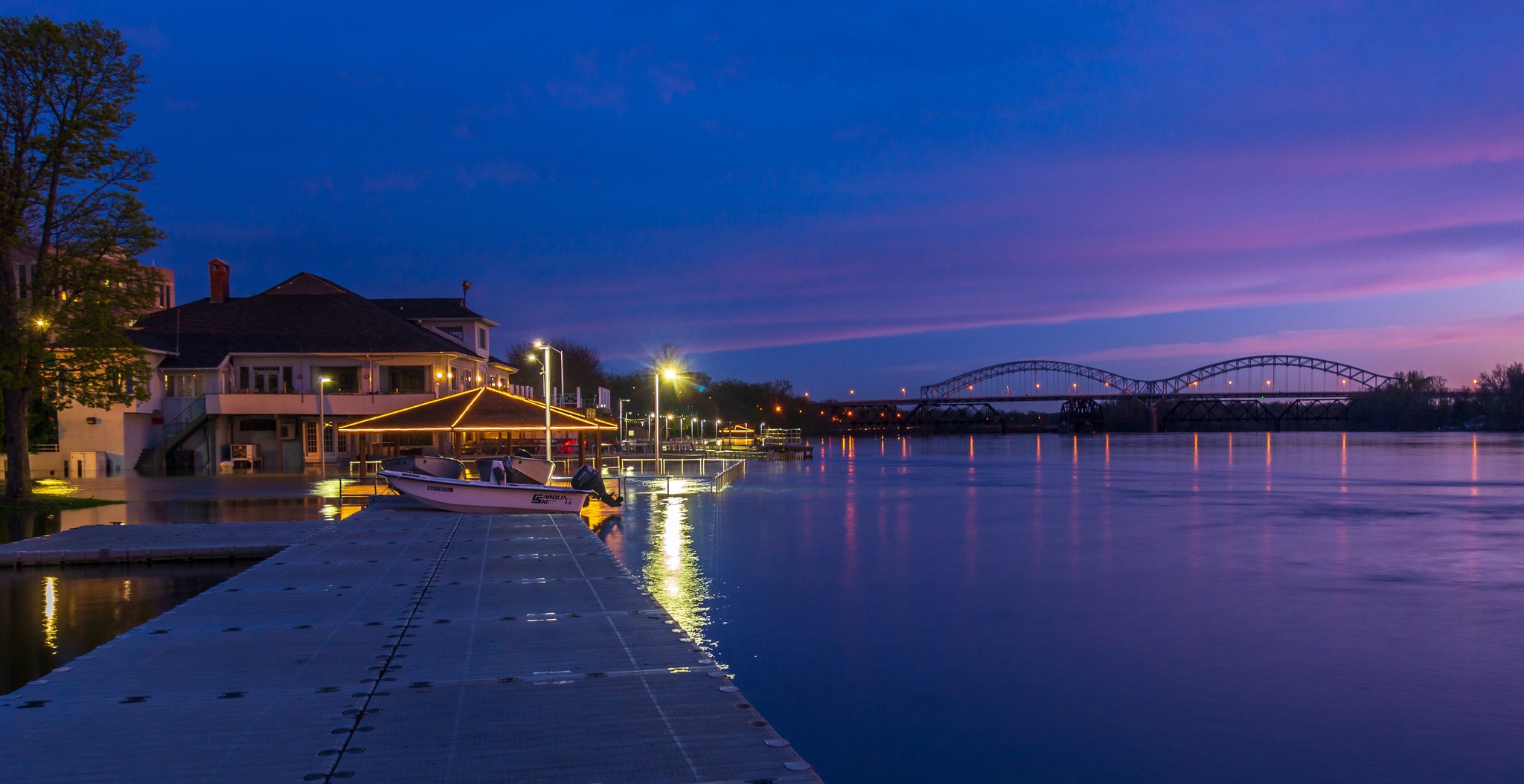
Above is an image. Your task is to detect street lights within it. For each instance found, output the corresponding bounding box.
[1194,382,1197,392]
[654,368,678,478]
[1036,384,1040,396]
[691,418,697,440]
[648,413,655,440]
[1229,380,1232,393]
[1342,380,1346,392]
[626,412,631,439]
[619,399,630,443]
[319,377,332,479]
[1007,385,1009,397]
[680,418,685,441]
[701,419,706,439]
[1073,384,1076,392]
[666,415,672,441]
[761,422,765,436]
[902,388,905,397]
[538,345,564,397]
[715,420,720,439]
[1267,380,1270,392]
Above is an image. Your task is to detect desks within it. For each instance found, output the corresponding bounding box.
[460,437,579,456]
[591,440,691,453]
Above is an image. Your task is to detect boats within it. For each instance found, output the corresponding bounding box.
[757,427,802,446]
[376,449,624,518]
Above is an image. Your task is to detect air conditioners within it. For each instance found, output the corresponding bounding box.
[280,424,297,440]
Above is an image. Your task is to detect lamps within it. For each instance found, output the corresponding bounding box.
[87,416,97,425]
